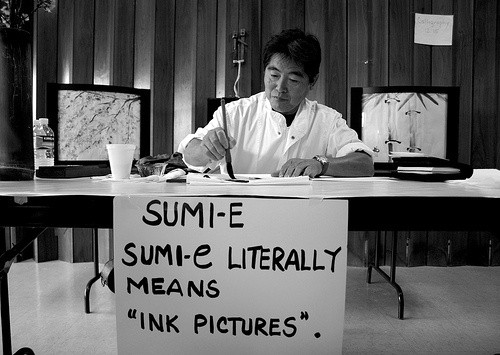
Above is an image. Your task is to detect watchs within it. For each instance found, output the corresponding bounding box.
[313,154,329,176]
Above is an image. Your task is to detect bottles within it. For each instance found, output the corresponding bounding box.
[33,119,41,179]
[36,118,54,166]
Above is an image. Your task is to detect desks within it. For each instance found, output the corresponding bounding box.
[0,161,500,355]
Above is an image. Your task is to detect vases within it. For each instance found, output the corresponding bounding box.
[0,28,36,180]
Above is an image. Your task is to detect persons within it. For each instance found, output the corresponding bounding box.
[177,28,376,178]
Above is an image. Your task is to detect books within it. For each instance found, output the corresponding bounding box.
[390,152,461,174]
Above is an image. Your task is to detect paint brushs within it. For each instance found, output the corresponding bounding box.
[220,97,235,181]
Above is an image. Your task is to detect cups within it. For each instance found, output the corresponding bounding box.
[135,162,168,177]
[106,144,136,180]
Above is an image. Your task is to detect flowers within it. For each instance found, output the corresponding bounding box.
[0,0,57,33]
